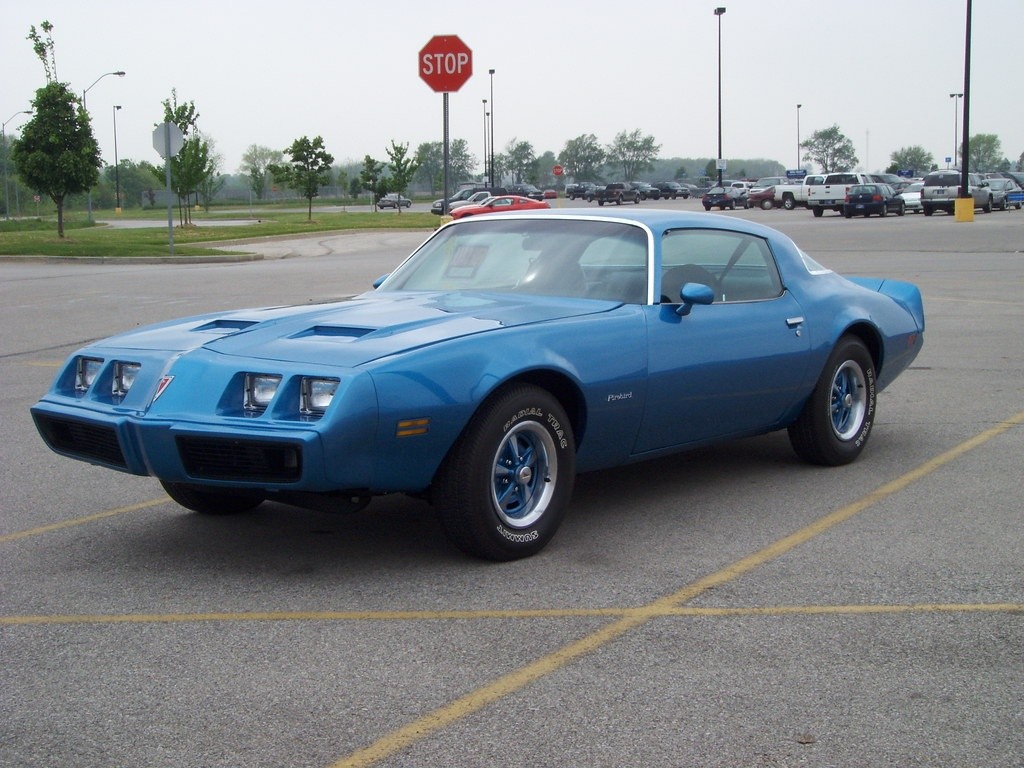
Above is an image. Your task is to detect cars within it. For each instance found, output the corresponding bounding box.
[508,185,545,202]
[477,197,512,207]
[543,189,558,198]
[449,196,549,220]
[377,193,412,209]
[29,209,926,565]
[431,187,508,216]
[566,172,1024,219]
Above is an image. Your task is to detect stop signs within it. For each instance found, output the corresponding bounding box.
[418,35,474,92]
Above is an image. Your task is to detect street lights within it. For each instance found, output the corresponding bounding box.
[482,69,495,188]
[950,93,963,166]
[2,111,33,220]
[713,7,727,188]
[797,104,802,170]
[113,106,123,214]
[82,71,126,221]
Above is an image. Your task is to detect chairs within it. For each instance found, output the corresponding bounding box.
[662,263,723,303]
[514,242,585,298]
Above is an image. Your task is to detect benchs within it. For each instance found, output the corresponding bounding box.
[587,271,777,303]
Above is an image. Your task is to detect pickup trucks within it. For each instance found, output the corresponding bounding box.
[807,173,876,217]
[594,182,641,206]
[448,192,491,210]
[774,175,827,210]
